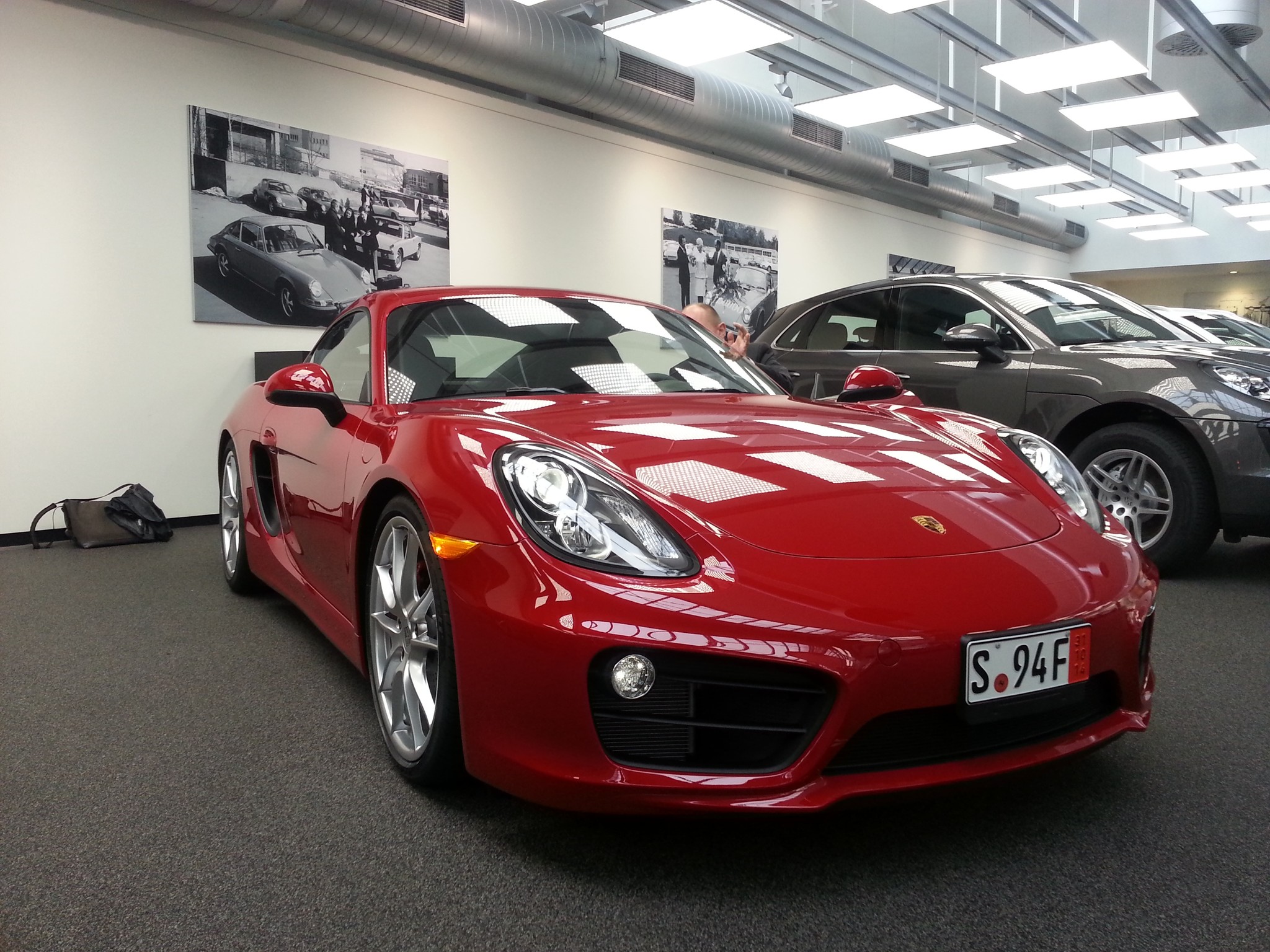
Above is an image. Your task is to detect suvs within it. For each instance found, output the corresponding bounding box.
[742,275,1270,577]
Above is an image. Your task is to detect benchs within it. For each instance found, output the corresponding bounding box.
[813,323,875,350]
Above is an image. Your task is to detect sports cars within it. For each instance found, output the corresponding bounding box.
[217,283,1163,821]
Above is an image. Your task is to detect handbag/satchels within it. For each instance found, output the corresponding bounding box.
[62,483,173,549]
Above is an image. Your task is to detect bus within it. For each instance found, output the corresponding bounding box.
[724,242,778,274]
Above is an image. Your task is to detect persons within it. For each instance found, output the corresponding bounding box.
[677,234,728,309]
[670,303,795,394]
[324,184,381,286]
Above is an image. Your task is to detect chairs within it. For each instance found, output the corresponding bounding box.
[279,230,298,251]
[899,302,944,350]
[379,223,402,238]
[251,235,276,252]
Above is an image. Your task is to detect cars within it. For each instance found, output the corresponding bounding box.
[371,196,419,226]
[252,179,308,219]
[663,240,730,268]
[296,187,347,225]
[331,174,449,241]
[1051,303,1270,357]
[352,216,423,272]
[710,265,774,340]
[207,215,378,324]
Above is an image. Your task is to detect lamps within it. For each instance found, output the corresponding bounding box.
[579,2,610,25]
[775,73,793,99]
